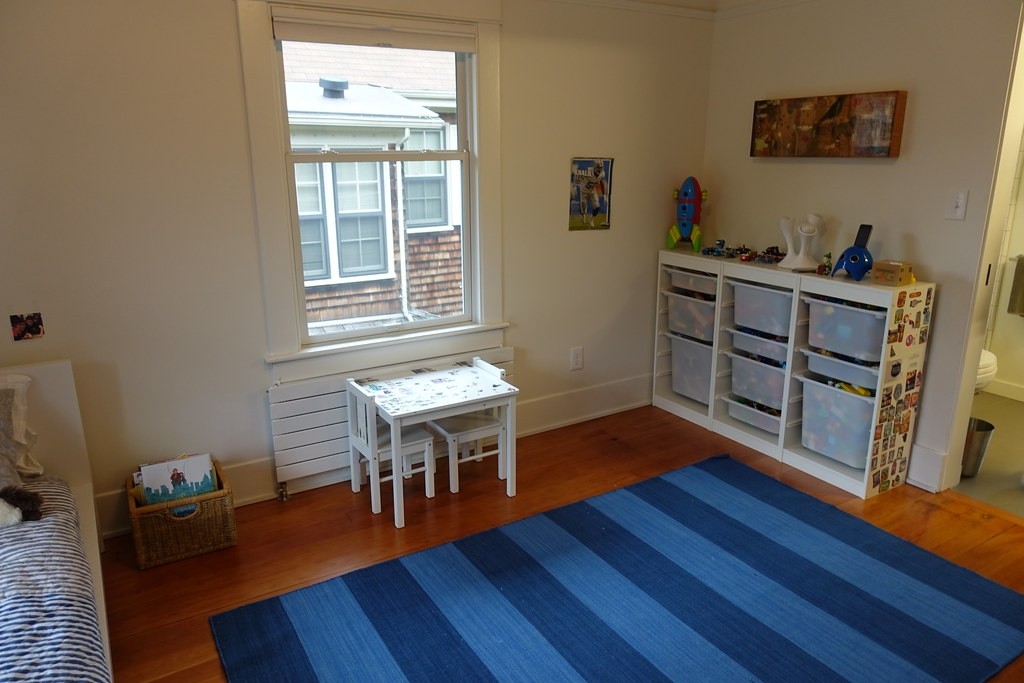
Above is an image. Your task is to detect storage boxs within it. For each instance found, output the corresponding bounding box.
[722,394,782,435]
[872,258,913,287]
[727,327,788,362]
[664,267,718,295]
[666,332,713,406]
[796,372,876,469]
[802,295,886,363]
[800,349,878,389]
[726,348,785,409]
[725,280,793,337]
[662,287,716,342]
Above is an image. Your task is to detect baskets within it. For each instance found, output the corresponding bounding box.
[126,459,236,569]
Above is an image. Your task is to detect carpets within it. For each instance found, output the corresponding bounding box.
[210,452,1024,683]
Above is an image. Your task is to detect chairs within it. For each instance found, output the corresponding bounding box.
[425,357,508,494]
[348,377,436,514]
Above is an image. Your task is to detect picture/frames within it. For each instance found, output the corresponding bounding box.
[750,90,908,158]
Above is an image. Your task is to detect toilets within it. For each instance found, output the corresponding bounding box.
[975,349,998,392]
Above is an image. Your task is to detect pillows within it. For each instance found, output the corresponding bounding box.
[0,374,46,481]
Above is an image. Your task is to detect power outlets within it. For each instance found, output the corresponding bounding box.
[570,346,583,370]
[944,189,969,220]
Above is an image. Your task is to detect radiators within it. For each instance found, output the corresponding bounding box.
[267,346,515,483]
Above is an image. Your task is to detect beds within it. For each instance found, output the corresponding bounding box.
[0,361,116,683]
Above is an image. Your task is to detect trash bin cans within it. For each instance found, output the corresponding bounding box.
[961,418,995,478]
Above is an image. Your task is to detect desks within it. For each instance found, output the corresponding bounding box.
[355,360,520,529]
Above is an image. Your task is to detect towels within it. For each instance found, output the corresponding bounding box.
[1007,255,1024,319]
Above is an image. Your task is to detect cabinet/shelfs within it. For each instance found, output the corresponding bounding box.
[651,249,936,499]
